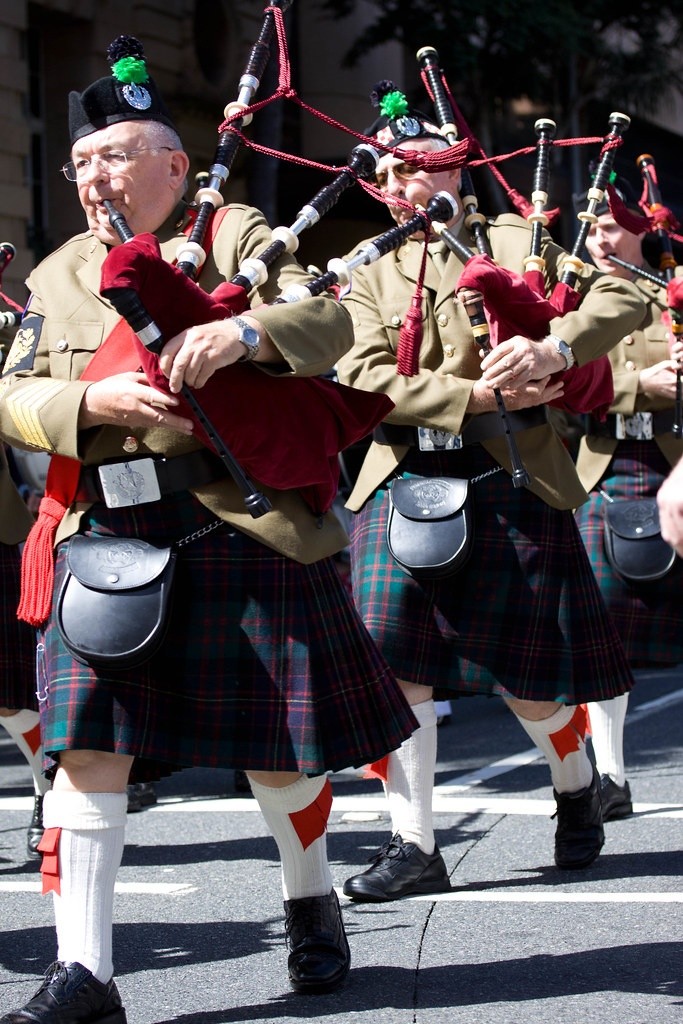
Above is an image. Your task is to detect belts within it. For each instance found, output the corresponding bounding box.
[373,403,547,451]
[73,449,234,509]
[585,406,683,440]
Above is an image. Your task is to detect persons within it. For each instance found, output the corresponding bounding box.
[569,178,683,824]
[334,109,646,909]
[0,67,355,1024]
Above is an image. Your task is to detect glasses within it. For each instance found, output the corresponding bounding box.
[59,146,172,182]
[367,161,420,189]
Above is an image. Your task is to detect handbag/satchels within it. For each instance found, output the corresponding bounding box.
[55,534,176,672]
[603,499,676,580]
[386,476,473,580]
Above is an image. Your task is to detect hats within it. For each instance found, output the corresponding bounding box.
[363,80,451,158]
[68,36,182,152]
[572,161,645,226]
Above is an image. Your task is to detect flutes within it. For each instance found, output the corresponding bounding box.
[101,0,681,521]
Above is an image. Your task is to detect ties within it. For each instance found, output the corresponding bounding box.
[426,240,448,279]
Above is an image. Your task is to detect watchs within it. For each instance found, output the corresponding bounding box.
[545,333,576,370]
[225,312,261,364]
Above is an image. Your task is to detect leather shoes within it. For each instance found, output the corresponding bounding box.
[27,793,45,859]
[600,772,633,822]
[343,830,451,901]
[0,961,128,1024]
[283,886,351,995]
[550,760,604,871]
[127,783,156,812]
